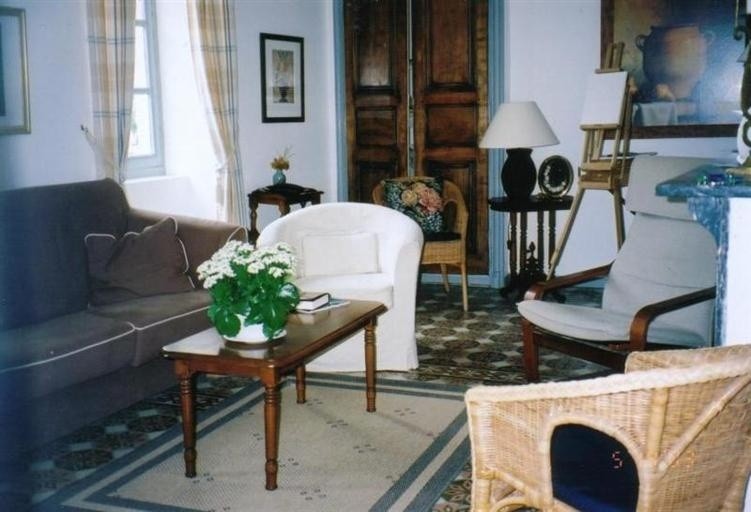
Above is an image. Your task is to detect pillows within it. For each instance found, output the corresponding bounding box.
[381,177,443,240]
[85,218,195,307]
[301,232,380,275]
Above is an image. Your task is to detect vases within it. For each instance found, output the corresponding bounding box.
[222,312,286,344]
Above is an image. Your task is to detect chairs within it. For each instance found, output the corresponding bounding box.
[373,175,469,315]
[516,157,738,382]
[465,343,751,511]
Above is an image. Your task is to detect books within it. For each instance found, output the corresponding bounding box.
[291,293,347,314]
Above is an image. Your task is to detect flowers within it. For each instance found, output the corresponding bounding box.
[196,241,300,342]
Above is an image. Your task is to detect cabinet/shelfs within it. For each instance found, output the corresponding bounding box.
[655,163,751,346]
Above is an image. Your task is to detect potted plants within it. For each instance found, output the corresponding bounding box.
[271,156,289,183]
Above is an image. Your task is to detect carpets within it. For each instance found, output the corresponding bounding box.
[32,370,473,512]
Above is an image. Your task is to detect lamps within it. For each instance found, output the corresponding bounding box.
[479,101,560,201]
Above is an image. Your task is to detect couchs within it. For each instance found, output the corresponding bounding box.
[1,178,246,464]
[254,201,424,374]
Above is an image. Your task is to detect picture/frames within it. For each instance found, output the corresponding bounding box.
[598,1,750,141]
[258,31,305,122]
[0,6,32,134]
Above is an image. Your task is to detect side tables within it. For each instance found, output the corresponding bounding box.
[247,190,324,232]
[487,194,575,303]
